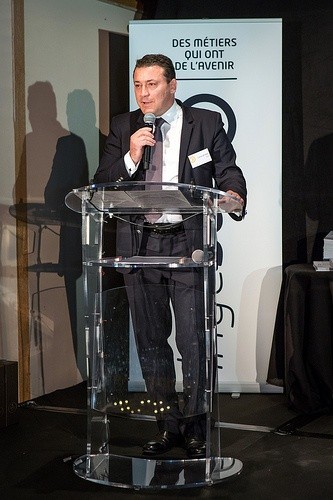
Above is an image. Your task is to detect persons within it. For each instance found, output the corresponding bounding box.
[91,53,247,458]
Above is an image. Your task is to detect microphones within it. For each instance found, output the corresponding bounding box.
[144,112,155,169]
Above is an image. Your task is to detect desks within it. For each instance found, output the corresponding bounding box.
[266,264,333,414]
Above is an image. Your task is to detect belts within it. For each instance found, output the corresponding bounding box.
[143,222,184,234]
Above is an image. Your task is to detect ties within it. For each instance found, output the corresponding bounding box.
[145,117,165,224]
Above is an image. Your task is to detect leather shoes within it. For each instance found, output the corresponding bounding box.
[143,430,177,455]
[184,435,211,458]
